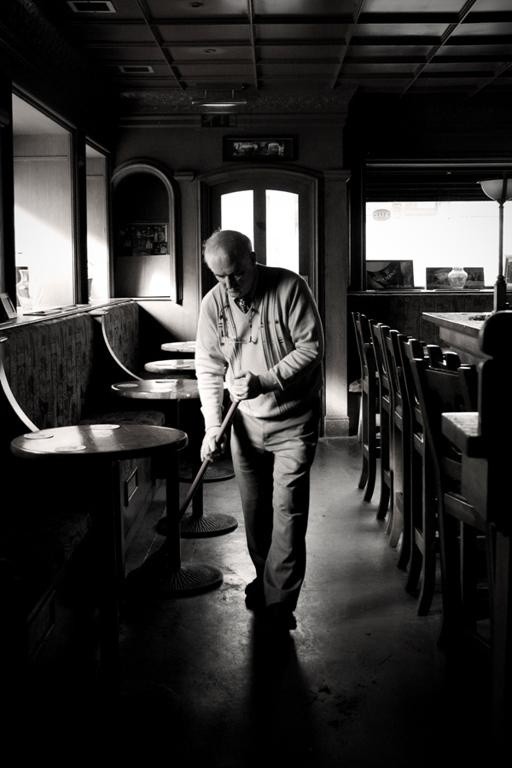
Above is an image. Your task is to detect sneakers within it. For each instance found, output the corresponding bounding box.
[268,604,297,629]
[246,577,265,598]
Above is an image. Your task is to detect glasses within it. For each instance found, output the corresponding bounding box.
[217,304,255,344]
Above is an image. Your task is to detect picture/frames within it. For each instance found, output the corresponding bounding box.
[114,222,168,257]
[362,256,512,292]
[222,133,299,163]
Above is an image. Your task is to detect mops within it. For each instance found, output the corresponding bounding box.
[128,399,239,647]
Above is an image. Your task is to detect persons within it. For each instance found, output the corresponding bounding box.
[193,229,325,639]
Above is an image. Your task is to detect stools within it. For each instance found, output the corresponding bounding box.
[350,309,512,696]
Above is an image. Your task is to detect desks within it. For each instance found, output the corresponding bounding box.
[145,358,238,540]
[9,420,189,691]
[118,378,229,602]
[160,340,235,483]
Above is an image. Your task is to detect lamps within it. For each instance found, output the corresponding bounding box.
[468,175,512,323]
[189,88,248,109]
